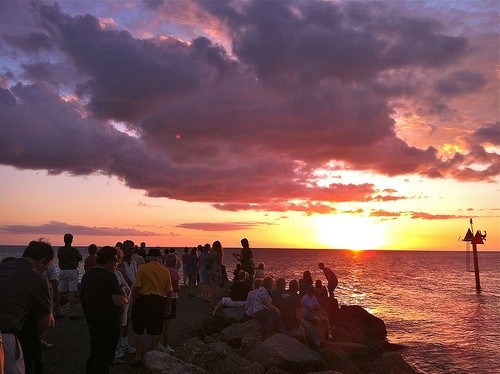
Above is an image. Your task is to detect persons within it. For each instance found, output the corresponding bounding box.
[0,234,338,374]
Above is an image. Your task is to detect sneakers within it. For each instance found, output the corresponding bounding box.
[151,346,174,356]
[113,344,136,365]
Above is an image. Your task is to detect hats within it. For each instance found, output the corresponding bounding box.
[120,241,135,250]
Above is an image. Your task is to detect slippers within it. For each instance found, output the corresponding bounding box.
[58,314,65,319]
[70,314,83,320]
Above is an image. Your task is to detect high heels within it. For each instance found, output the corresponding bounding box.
[41,340,53,350]
